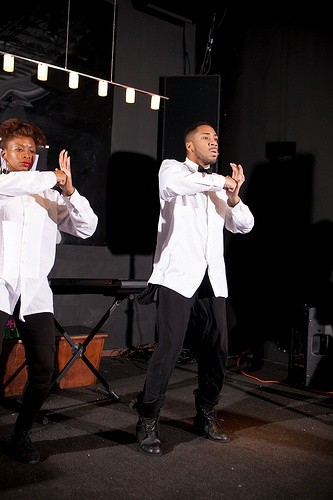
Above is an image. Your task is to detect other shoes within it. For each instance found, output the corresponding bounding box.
[12,436,40,465]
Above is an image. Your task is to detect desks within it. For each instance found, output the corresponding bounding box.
[44,276,157,400]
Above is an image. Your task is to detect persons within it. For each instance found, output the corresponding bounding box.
[0,116,98,464]
[133,121,255,455]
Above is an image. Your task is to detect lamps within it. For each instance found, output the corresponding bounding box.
[0,0,170,110]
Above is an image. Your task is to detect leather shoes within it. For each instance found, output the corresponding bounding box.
[135,414,162,457]
[194,409,230,443]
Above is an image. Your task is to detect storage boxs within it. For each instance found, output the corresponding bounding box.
[52,325,111,390]
[0,339,28,397]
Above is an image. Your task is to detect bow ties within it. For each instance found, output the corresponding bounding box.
[198,164,213,174]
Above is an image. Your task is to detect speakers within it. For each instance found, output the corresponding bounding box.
[158,73,220,175]
[104,150,159,256]
